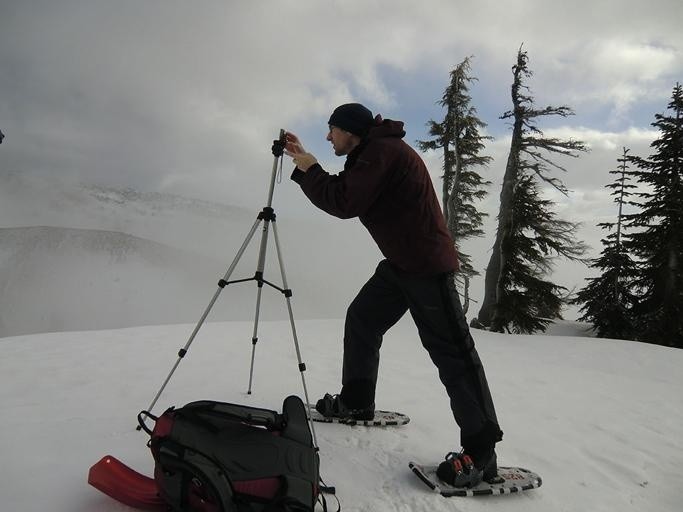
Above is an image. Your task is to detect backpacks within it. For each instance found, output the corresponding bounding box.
[137,395,340,512]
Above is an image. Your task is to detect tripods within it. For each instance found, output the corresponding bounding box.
[136,140,321,452]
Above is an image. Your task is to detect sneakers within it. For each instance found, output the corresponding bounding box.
[436,447,497,489]
[316,393,375,421]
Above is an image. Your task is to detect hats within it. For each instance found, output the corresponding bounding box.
[328,103,373,136]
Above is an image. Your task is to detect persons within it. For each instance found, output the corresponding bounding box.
[285,104,502,488]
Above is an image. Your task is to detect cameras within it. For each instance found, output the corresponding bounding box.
[279,129,287,142]
